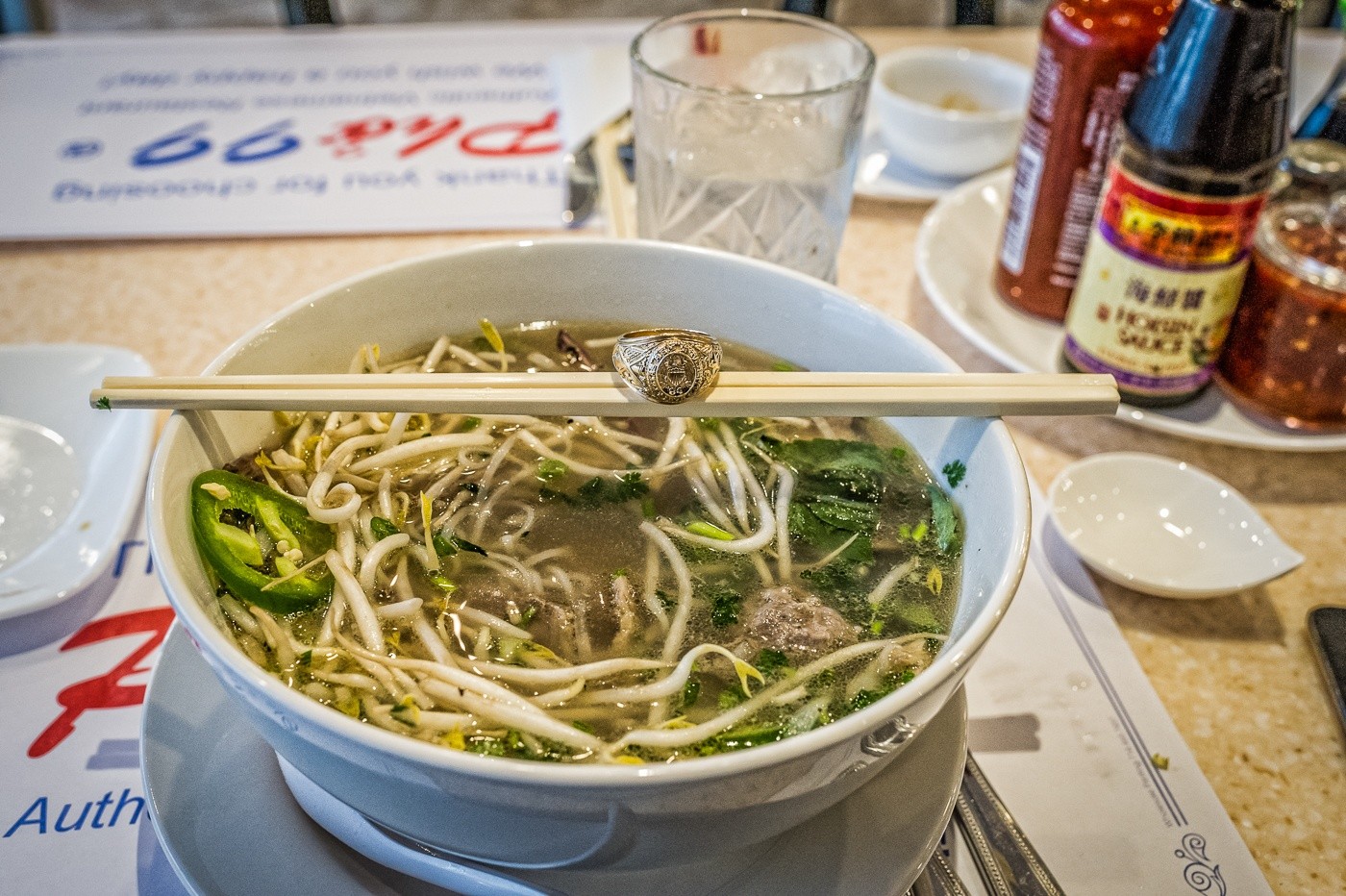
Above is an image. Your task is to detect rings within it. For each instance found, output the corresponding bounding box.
[611,328,722,406]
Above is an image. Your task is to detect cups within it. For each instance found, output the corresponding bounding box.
[630,8,876,286]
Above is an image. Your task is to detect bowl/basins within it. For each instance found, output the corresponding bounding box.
[156,233,1031,891]
[867,47,1032,179]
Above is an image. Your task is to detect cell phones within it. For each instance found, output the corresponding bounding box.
[1302,603,1345,732]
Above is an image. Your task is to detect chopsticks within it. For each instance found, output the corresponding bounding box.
[90,361,1122,419]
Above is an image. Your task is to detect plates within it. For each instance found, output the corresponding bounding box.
[1047,453,1304,602]
[3,340,157,616]
[913,166,1344,454]
[137,611,966,893]
[850,130,966,206]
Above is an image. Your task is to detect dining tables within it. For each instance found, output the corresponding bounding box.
[0,20,1342,893]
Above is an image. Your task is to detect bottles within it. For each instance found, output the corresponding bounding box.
[1000,0,1341,437]
